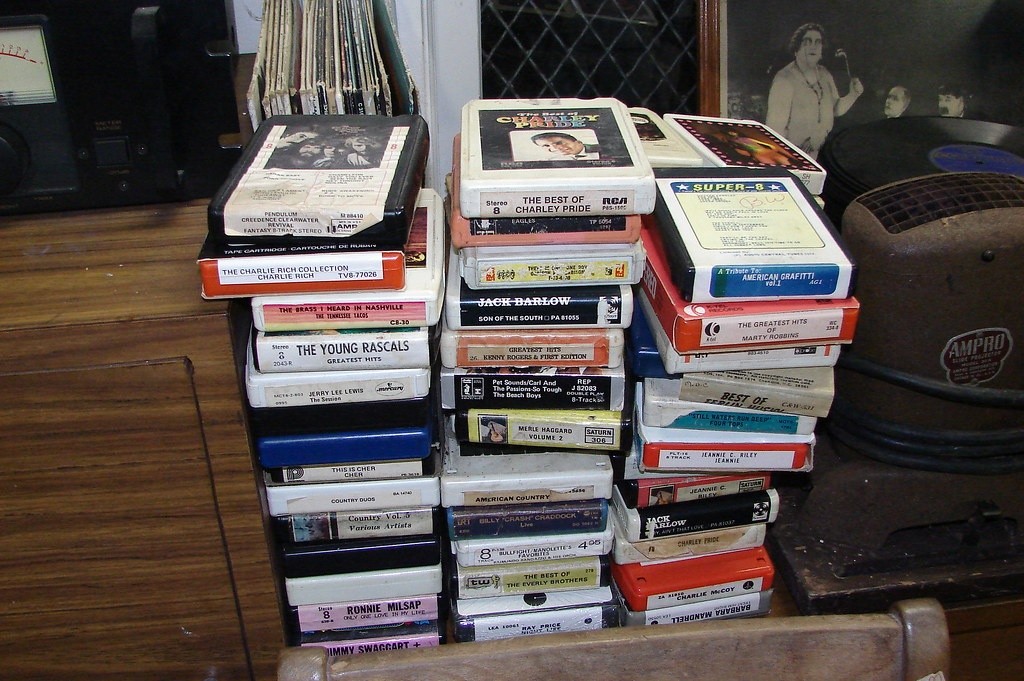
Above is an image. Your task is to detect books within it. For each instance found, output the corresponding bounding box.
[245,0,392,135]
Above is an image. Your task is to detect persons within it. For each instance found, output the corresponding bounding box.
[938,80,987,120]
[274,122,382,167]
[721,125,795,165]
[884,86,914,118]
[531,133,600,160]
[764,22,863,161]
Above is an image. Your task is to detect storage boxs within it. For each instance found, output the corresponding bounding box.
[198,96,863,654]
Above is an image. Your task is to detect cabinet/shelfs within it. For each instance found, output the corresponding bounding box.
[0,49,293,680]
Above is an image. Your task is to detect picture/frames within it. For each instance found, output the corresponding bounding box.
[699,0,1024,160]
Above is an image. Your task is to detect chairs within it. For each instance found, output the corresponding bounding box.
[279,597,951,681]
